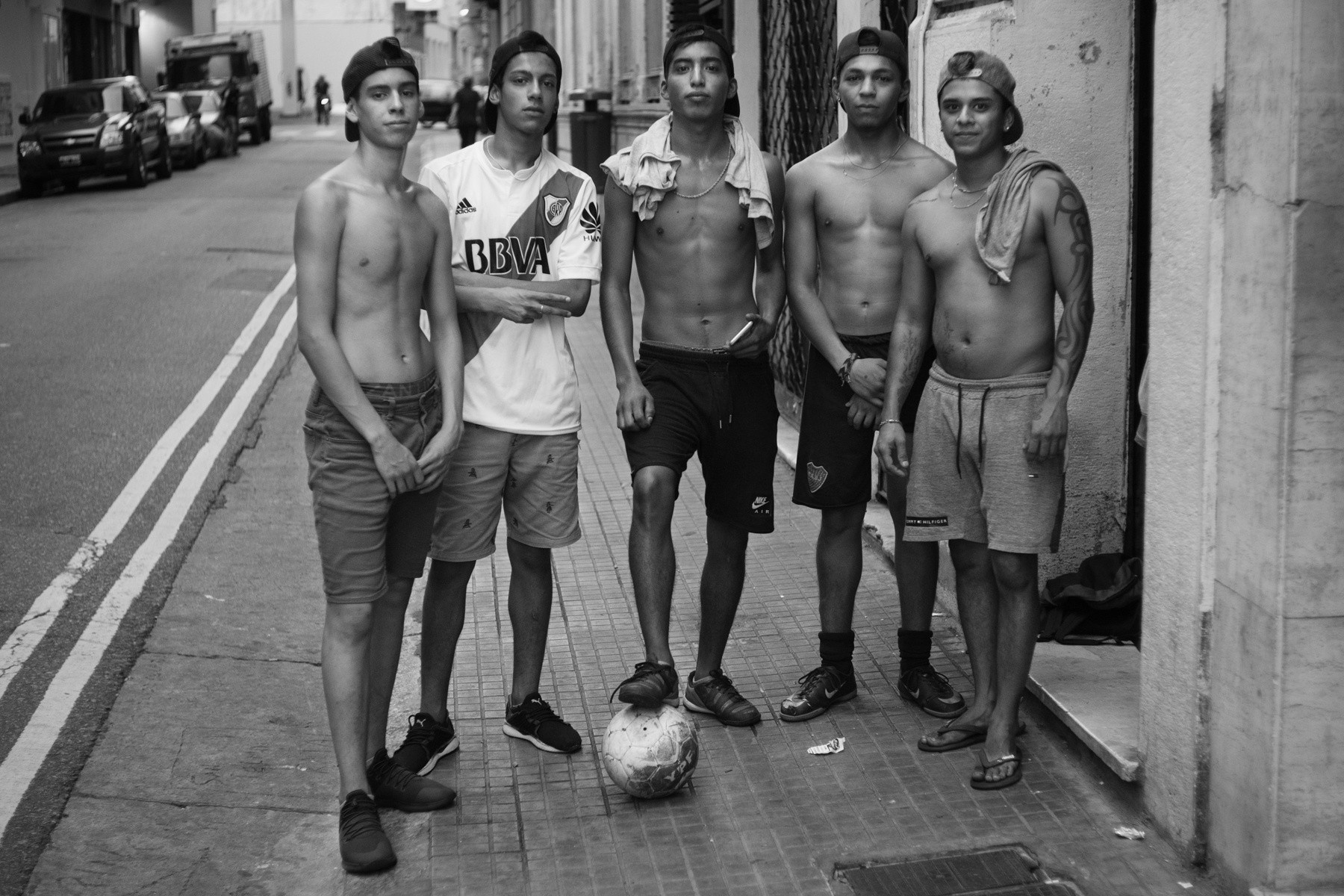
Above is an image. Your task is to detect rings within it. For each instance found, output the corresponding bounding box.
[539,304,543,312]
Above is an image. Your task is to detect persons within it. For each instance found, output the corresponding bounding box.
[602,24,787,727]
[313,75,333,124]
[295,36,464,873]
[781,28,966,721]
[448,76,483,149]
[220,74,241,155]
[873,50,1095,790]
[390,30,602,778]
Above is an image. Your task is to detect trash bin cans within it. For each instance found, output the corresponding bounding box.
[565,87,615,194]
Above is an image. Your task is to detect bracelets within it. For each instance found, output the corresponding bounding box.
[837,352,859,386]
[877,419,901,428]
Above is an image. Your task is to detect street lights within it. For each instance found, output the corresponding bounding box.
[420,19,490,83]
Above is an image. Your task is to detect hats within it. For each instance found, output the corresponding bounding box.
[936,50,1023,146]
[480,36,561,134]
[834,27,907,114]
[661,23,741,118]
[341,37,419,142]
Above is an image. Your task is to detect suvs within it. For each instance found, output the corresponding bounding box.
[17,76,172,192]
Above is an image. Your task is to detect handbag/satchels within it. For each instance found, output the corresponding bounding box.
[1040,550,1141,644]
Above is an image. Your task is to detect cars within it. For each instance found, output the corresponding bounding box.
[419,79,491,129]
[149,88,239,164]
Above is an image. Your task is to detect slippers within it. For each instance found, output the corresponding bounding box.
[969,746,1022,788]
[918,718,1026,751]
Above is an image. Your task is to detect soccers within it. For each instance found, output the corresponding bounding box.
[600,701,700,802]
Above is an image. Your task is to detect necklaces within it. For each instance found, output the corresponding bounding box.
[669,136,735,199]
[483,139,543,181]
[840,134,909,179]
[946,171,995,209]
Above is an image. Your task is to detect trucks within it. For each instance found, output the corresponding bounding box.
[154,28,273,146]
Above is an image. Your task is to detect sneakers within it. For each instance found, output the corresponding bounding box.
[899,664,967,717]
[501,691,582,753]
[610,660,679,707]
[339,789,396,870]
[391,709,459,776]
[778,665,857,719]
[366,748,457,811]
[682,669,761,725]
